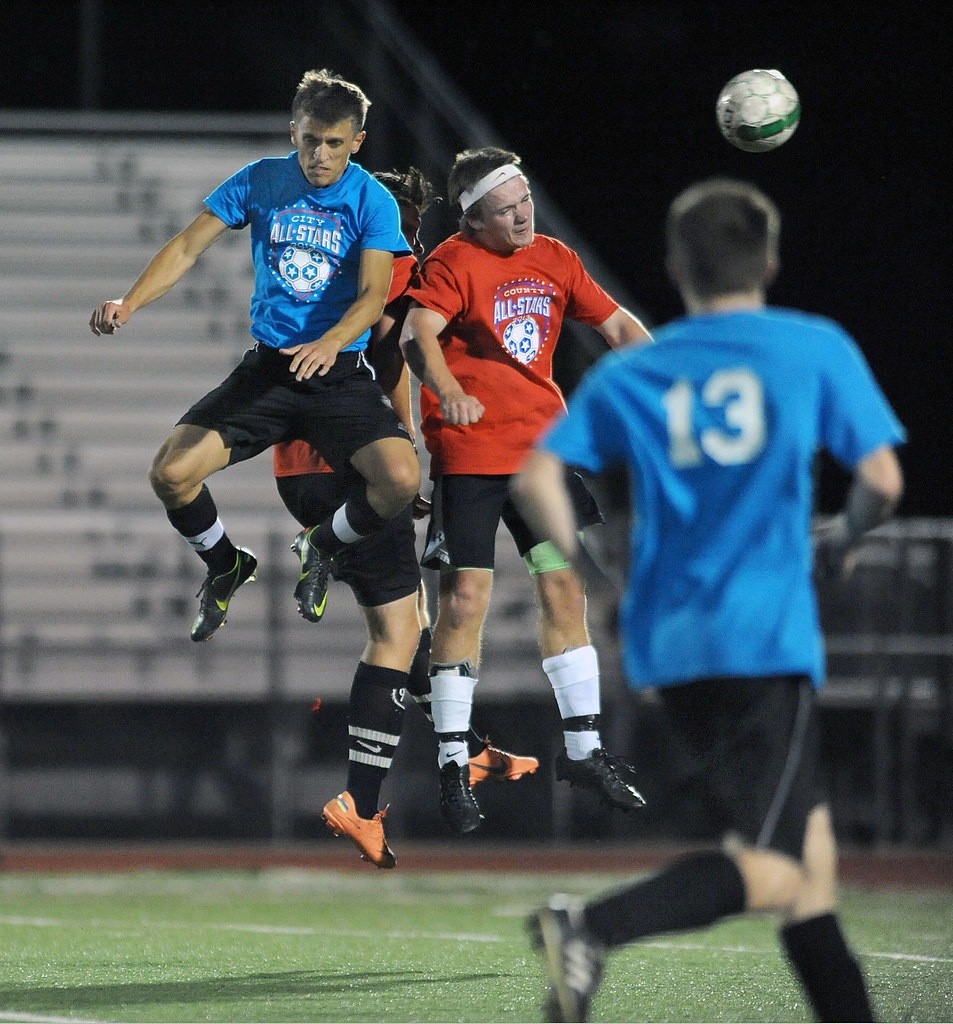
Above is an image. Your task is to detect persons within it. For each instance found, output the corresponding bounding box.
[400,146,664,830]
[89,70,418,641]
[509,184,904,1023]
[272,168,538,870]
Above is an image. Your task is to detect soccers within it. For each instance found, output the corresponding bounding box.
[717,69,802,152]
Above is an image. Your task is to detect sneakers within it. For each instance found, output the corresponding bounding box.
[319,745,647,871]
[526,904,606,1023]
[189,523,333,643]
